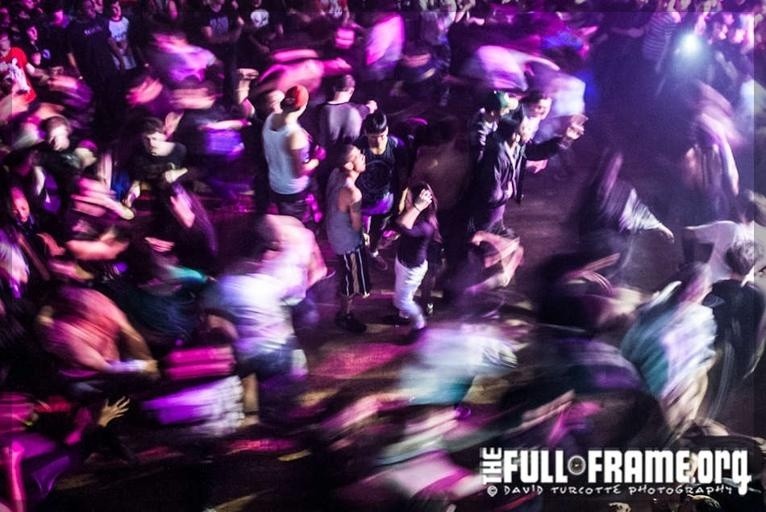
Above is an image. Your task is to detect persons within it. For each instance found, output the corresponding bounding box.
[1,1,766,512]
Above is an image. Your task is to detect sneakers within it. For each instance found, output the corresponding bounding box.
[383,314,410,325]
[368,251,387,271]
[403,326,426,345]
[335,311,355,330]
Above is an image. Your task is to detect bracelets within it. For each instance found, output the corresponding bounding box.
[413,203,421,212]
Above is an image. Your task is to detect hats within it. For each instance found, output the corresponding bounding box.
[284,83,310,108]
[485,92,520,113]
